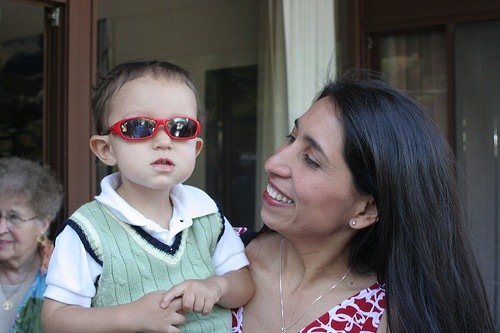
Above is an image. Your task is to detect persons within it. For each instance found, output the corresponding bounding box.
[1,158,63,332]
[229,77,496,332]
[41,61,256,333]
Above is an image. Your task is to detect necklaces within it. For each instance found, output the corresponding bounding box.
[279,236,355,332]
[0,252,38,311]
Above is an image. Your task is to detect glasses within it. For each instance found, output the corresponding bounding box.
[1,211,40,227]
[94,114,201,166]
